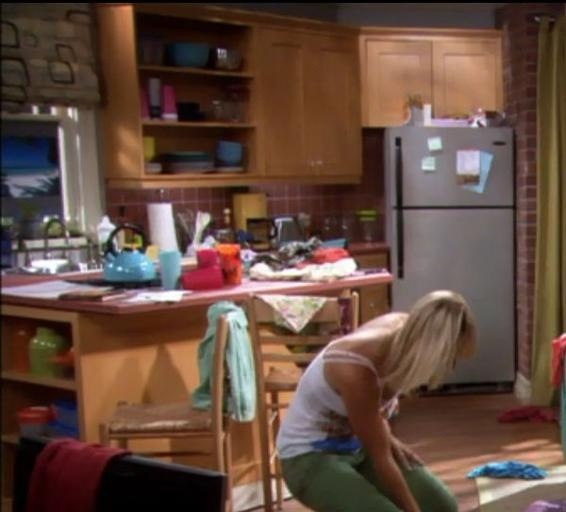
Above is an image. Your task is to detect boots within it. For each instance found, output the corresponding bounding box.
[182,250,225,289]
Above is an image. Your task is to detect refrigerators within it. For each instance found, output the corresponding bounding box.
[383,120,522,396]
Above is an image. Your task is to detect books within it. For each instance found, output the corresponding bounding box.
[60,290,126,302]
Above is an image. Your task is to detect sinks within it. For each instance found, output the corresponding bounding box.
[23,257,104,273]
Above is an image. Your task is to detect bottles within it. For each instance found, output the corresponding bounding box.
[354,209,379,243]
[12,321,69,379]
[96,214,118,262]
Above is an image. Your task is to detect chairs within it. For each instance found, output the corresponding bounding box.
[239,285,363,511]
[96,312,241,510]
[7,427,233,512]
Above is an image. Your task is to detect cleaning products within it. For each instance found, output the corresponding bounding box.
[97,215,119,254]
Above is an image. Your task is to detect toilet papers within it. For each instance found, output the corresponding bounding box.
[145,201,180,253]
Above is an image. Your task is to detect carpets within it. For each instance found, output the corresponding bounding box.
[473,466,564,512]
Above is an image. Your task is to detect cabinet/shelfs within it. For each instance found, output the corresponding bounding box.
[94,1,250,191]
[247,10,365,184]
[355,26,506,129]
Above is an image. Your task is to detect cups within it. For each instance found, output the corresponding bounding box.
[218,243,244,287]
[158,252,183,290]
[142,78,180,120]
[182,264,223,290]
[320,215,355,242]
[195,249,219,268]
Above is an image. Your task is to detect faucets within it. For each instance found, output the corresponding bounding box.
[42,216,70,256]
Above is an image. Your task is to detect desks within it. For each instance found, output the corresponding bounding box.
[0,264,390,510]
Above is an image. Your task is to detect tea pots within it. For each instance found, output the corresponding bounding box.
[102,223,156,284]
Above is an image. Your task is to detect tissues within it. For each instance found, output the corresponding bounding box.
[180,208,218,277]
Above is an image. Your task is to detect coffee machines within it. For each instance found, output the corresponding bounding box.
[232,191,271,249]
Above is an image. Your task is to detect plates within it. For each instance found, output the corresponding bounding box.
[141,138,213,173]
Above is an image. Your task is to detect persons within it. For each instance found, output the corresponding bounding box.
[277,290,479,512]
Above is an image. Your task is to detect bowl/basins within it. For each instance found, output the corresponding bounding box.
[209,48,243,72]
[15,400,80,441]
[165,40,210,67]
[214,139,242,167]
